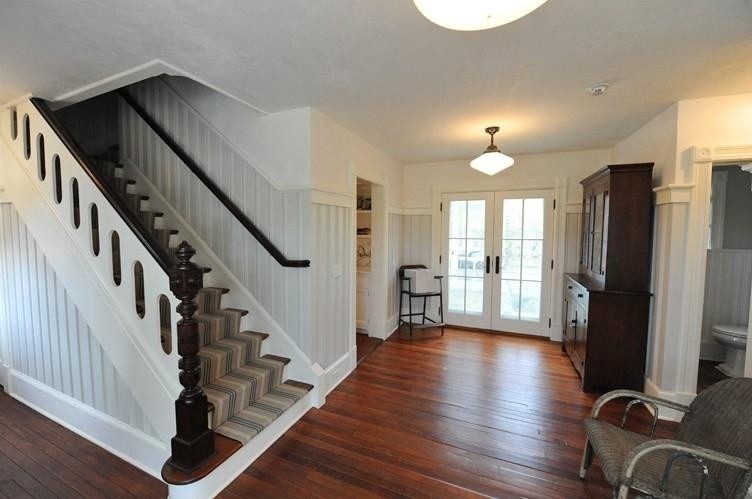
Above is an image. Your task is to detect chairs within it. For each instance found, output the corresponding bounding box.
[579,376,752,498]
[397,264,447,338]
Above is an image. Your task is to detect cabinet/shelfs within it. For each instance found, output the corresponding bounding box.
[356,181,373,335]
[561,162,656,395]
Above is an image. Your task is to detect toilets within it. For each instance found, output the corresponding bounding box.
[712,323,748,379]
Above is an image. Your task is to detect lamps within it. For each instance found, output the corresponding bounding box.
[467,126,515,177]
